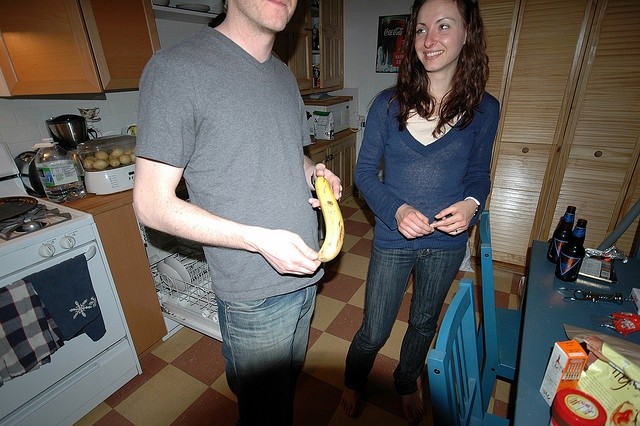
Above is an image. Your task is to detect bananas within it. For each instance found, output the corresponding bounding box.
[311,177,346,265]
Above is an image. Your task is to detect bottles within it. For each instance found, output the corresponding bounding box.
[547,206,576,265]
[34,138,87,205]
[555,219,588,283]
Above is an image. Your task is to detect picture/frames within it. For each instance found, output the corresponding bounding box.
[374,13,412,75]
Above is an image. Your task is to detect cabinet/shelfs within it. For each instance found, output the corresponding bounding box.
[488,1,639,272]
[59,202,168,360]
[288,1,345,96]
[474,0,520,261]
[334,128,357,204]
[79,0,162,91]
[615,156,640,257]
[0,0,104,97]
[304,140,343,206]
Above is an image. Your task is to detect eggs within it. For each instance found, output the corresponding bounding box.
[79,147,137,169]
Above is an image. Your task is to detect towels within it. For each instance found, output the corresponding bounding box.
[0,279,66,387]
[29,252,106,342]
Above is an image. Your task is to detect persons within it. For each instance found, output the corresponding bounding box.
[132,0,343,426]
[339,0,501,424]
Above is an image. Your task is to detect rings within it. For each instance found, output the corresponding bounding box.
[453,228,459,232]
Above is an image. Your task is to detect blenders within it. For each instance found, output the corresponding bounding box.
[46,114,94,150]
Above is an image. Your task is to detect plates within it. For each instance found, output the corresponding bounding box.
[153,264,184,292]
[165,256,195,285]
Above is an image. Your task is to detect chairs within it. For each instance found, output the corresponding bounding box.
[427,277,512,426]
[477,210,523,411]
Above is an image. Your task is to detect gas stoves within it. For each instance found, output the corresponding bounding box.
[1,194,89,242]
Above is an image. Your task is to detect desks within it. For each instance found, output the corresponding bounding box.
[514,240,639,426]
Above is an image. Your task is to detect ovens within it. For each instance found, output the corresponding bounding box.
[0,218,143,425]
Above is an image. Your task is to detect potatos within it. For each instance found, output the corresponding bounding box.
[109,156,120,167]
[110,147,124,158]
[85,156,97,162]
[130,153,136,163]
[95,151,108,161]
[119,154,131,165]
[92,160,107,170]
[83,158,93,169]
[125,148,133,155]
